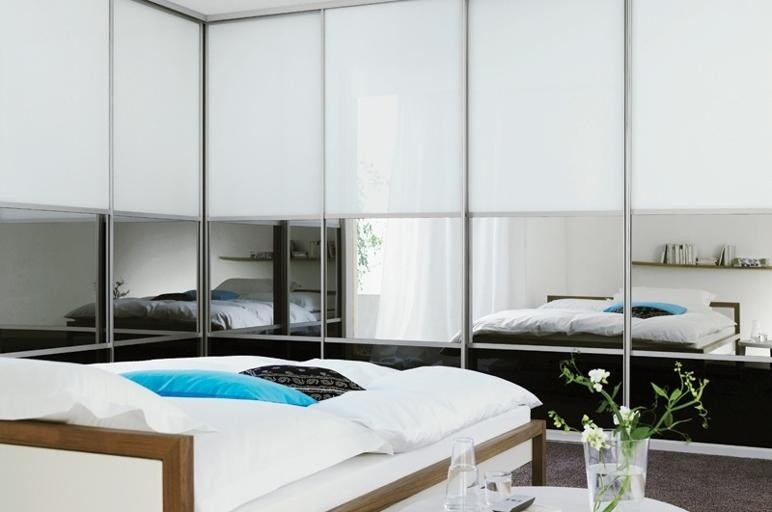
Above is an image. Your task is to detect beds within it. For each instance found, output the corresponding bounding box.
[0,359,547,511]
[443,294,742,354]
[63,277,335,332]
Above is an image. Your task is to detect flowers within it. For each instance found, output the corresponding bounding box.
[547,354,713,512]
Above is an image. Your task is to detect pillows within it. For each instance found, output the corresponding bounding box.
[239,365,367,403]
[120,369,319,408]
[151,288,240,302]
[1,359,191,435]
[604,285,714,318]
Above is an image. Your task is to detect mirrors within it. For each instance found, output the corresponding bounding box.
[206,207,770,359]
[0,200,201,358]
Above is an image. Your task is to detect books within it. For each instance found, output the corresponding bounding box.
[661,241,738,268]
[290,239,338,259]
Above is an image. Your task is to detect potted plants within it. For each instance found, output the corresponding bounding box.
[353,219,387,337]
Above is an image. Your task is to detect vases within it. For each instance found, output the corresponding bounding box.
[582,431,648,512]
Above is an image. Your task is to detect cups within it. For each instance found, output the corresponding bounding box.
[443,437,482,511]
[485,470,513,512]
